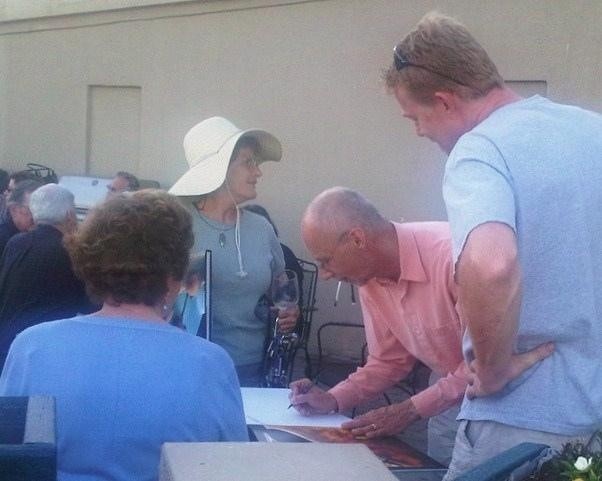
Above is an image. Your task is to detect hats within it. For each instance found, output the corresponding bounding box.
[168,115,282,202]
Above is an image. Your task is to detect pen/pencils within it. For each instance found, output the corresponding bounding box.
[287,367,324,410]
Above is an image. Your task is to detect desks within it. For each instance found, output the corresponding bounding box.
[247,417,450,481]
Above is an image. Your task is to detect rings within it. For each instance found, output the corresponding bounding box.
[371,423,377,431]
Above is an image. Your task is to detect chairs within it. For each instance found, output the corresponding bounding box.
[264,257,319,382]
[315,322,420,434]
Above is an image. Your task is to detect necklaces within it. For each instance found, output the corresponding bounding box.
[196,205,243,248]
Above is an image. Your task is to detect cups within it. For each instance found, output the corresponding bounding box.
[271,268,300,312]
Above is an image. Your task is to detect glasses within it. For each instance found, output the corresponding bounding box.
[232,152,259,169]
[393,47,466,88]
[106,184,116,193]
[314,232,350,271]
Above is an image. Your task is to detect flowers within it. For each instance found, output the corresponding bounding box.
[521,436,602,481]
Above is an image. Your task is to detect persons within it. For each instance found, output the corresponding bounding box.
[380,8,602,481]
[105,171,139,194]
[0,187,250,481]
[165,113,301,386]
[0,166,102,373]
[282,186,471,467]
